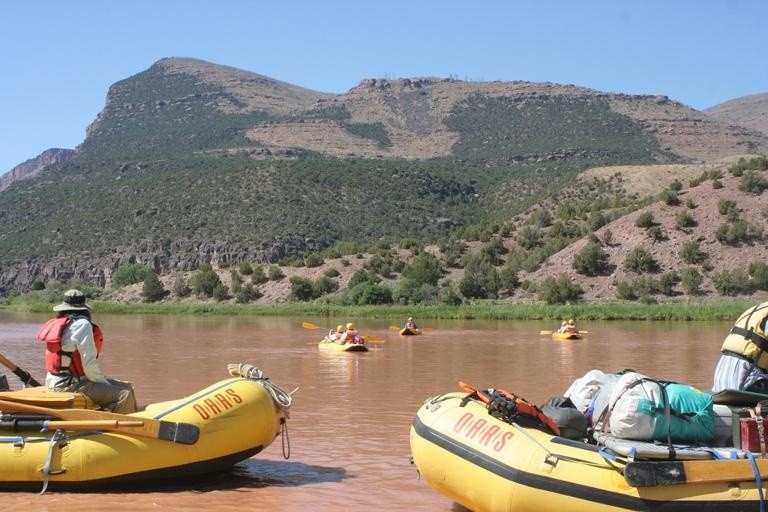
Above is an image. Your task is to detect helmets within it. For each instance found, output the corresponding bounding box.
[408,317,413,321]
[336,324,343,333]
[53,289,94,313]
[561,318,575,326]
[346,322,354,330]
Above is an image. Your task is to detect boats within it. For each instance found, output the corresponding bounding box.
[398,326,423,336]
[401,384,767,511]
[0,373,299,492]
[552,330,582,340]
[317,335,369,352]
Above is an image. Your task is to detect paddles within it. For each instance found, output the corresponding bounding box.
[389,327,432,331]
[0,400,199,445]
[540,331,589,335]
[303,323,386,343]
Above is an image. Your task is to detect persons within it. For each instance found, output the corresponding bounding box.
[34,290,137,415]
[561,319,577,334]
[405,317,416,331]
[558,321,568,333]
[338,322,359,345]
[712,300,768,395]
[326,325,345,343]
